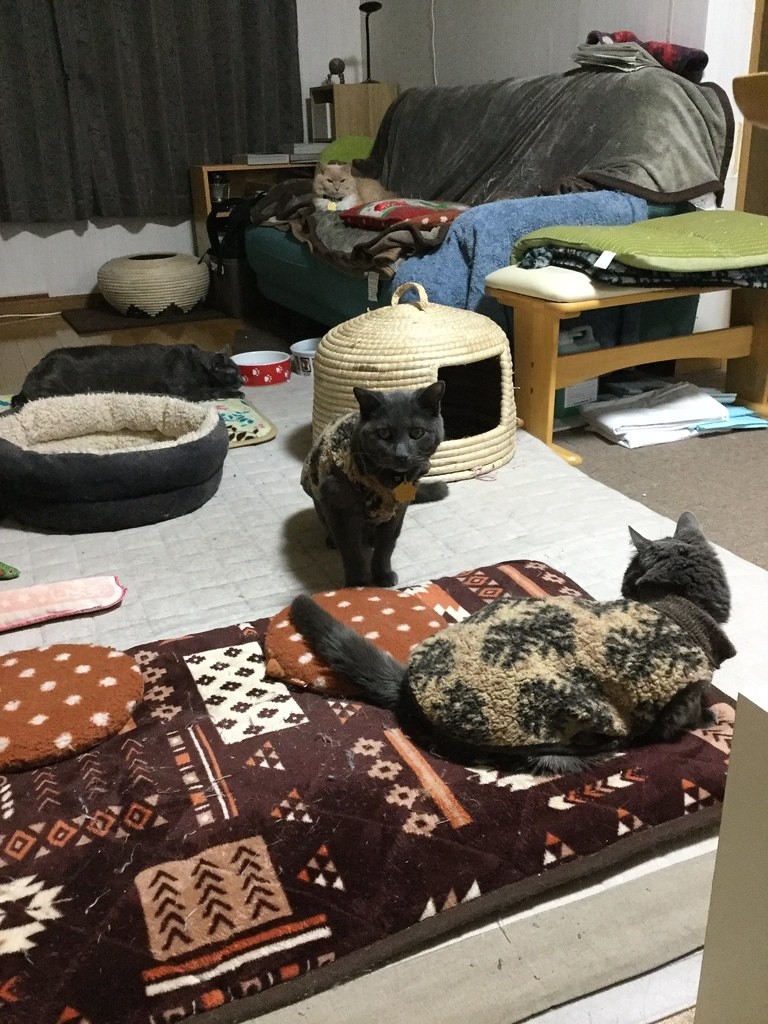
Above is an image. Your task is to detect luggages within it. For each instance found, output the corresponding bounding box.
[208,256,253,318]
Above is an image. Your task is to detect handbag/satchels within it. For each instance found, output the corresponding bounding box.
[198,198,249,278]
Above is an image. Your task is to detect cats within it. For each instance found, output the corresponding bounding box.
[311,160,391,209]
[301,379,449,586]
[287,511,730,771]
[11,344,246,408]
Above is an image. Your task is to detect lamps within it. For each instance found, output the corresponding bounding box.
[359,1,382,84]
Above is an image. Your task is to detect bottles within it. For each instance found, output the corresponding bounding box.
[554,326,598,418]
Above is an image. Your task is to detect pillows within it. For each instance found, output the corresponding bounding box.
[339,197,472,228]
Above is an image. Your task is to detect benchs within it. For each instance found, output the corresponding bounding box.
[484,286,768,465]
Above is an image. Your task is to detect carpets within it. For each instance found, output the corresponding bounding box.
[0,374,768,1024]
[61,304,230,335]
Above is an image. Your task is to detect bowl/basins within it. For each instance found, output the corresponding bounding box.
[228,351,291,386]
[289,338,322,376]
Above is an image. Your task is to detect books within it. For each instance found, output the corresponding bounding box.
[229,101,333,165]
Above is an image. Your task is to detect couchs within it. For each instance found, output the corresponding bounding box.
[246,64,735,324]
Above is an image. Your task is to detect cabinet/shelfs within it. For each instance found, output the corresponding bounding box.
[191,82,399,265]
